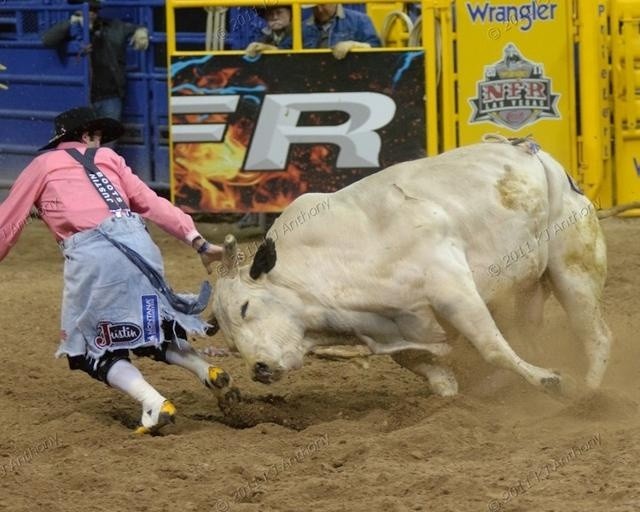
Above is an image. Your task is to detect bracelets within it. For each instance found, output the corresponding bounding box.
[198,241,209,253]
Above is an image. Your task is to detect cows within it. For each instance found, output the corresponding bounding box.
[204,139,613,397]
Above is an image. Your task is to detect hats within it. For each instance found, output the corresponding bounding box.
[46,24,70,64]
[36,105,124,150]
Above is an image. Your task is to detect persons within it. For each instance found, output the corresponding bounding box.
[253,4,292,47]
[43,0,149,151]
[246,2,381,60]
[0,107,259,436]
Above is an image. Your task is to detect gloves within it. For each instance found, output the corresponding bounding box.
[244,42,278,56]
[334,39,369,59]
[130,28,149,52]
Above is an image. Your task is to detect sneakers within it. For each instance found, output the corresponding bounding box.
[204,365,241,416]
[133,401,177,436]
